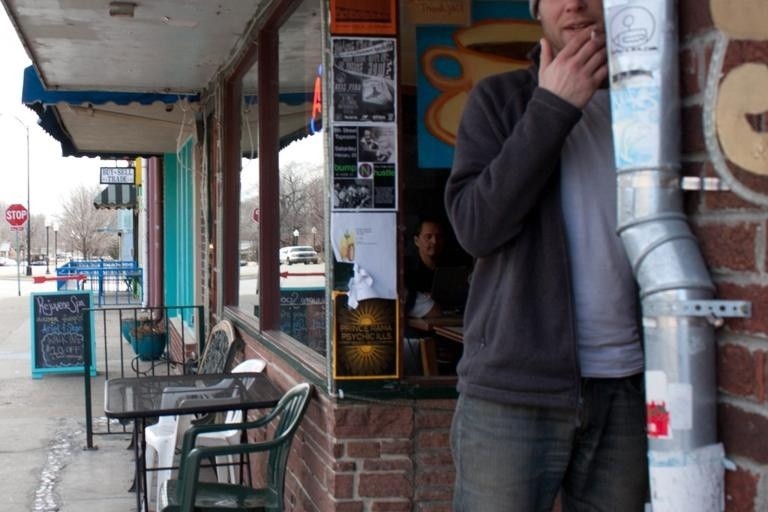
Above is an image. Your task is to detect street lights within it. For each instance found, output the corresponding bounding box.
[293,228,299,245]
[44,216,51,274]
[52,220,59,271]
[311,226,317,248]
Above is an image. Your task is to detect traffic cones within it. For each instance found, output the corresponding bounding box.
[5,204,28,225]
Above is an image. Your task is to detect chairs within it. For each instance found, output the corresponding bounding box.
[125,316,241,495]
[134,358,267,512]
[151,381,316,512]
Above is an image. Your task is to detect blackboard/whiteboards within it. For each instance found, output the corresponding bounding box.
[30,289,96,373]
[277,287,326,357]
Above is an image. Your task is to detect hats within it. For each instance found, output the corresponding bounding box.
[528,0,540,22]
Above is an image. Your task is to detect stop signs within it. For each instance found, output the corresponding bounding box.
[251,208,259,224]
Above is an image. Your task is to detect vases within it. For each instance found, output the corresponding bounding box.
[127,325,169,363]
[119,316,153,345]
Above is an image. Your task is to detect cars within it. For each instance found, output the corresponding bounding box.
[239,260,248,266]
[278,245,317,266]
[26,251,72,268]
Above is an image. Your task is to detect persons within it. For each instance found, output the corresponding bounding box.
[442,0,655,512]
[403,219,471,378]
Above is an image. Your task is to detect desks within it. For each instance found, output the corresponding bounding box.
[407,310,467,380]
[432,322,466,344]
[102,370,288,511]
